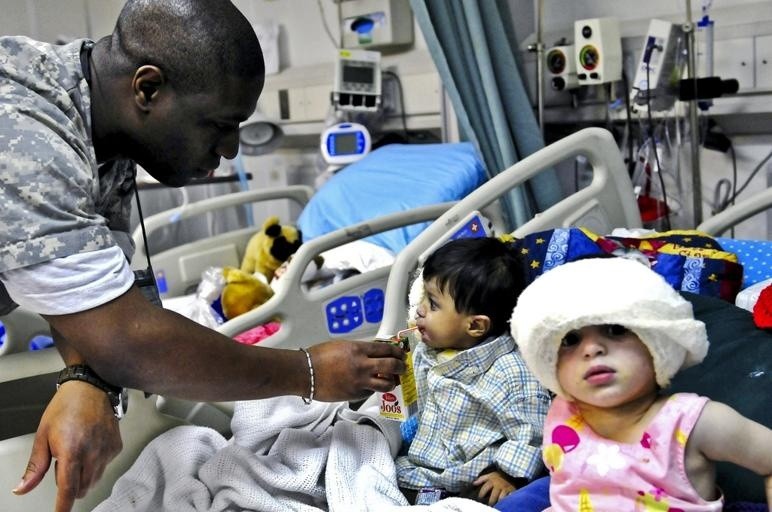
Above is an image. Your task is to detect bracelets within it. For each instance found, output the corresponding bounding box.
[299,342,316,407]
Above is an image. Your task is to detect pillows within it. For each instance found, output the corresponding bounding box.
[295,141,489,253]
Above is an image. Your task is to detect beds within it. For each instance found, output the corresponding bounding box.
[79,122,771,510]
[0,136,499,510]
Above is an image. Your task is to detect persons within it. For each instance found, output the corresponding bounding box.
[395,236,550,512]
[506,254,771,512]
[1,1,406,511]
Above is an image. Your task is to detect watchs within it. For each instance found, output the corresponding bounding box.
[56,364,129,420]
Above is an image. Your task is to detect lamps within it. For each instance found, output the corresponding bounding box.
[233,108,284,158]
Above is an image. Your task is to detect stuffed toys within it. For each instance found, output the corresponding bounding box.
[241,216,302,284]
[212,265,275,324]
